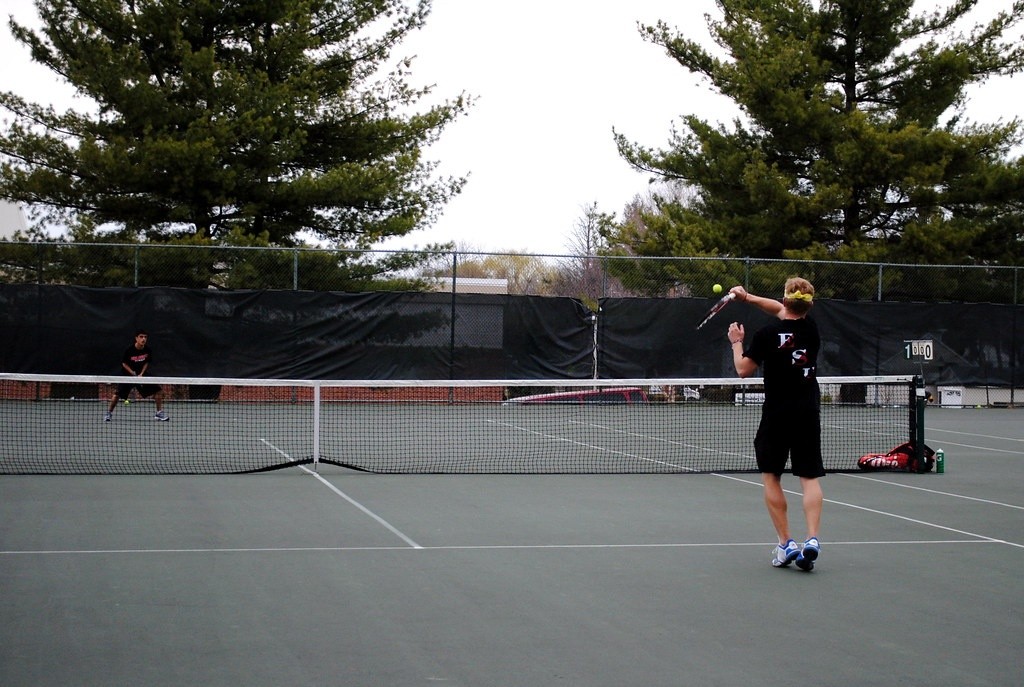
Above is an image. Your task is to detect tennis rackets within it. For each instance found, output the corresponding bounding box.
[697,291,738,331]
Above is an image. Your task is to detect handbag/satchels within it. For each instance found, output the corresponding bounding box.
[858,442,935,474]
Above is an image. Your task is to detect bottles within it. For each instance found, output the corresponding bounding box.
[936,448,944,474]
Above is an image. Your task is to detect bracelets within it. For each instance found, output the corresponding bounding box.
[730,339,744,345]
[741,292,747,303]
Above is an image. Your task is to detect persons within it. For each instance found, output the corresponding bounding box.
[104,330,170,421]
[727,276,828,572]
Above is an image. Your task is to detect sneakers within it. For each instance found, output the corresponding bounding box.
[795,537,820,571]
[155,410,169,421]
[771,539,801,567]
[105,411,112,421]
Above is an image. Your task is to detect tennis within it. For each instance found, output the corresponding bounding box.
[125,400,129,404]
[713,284,723,294]
